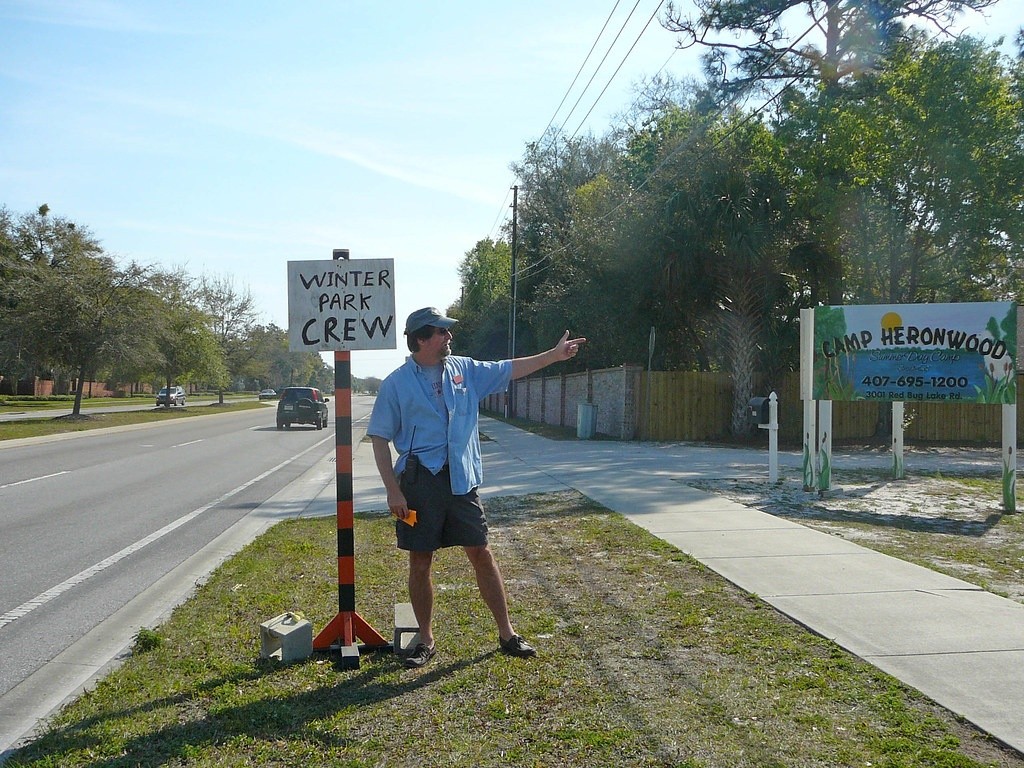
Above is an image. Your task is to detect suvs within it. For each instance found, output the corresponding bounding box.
[155,385,187,407]
[275,386,330,431]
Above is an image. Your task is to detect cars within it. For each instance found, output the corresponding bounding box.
[258,388,277,400]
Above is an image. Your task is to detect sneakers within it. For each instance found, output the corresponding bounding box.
[500,635,537,658]
[405,643,436,667]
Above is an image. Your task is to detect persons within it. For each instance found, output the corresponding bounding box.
[367,308,587,667]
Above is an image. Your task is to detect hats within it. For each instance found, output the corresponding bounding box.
[403,306,460,336]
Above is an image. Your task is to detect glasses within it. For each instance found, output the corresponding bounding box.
[436,329,451,335]
